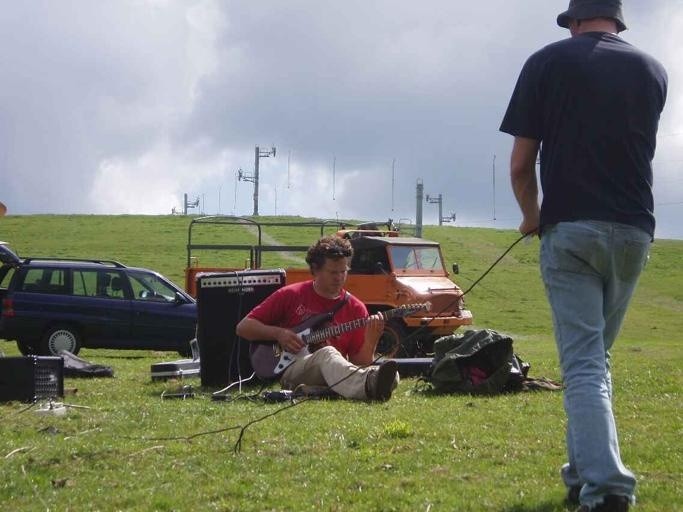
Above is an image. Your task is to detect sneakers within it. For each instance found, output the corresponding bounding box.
[366,360,398,401]
[569,485,628,511]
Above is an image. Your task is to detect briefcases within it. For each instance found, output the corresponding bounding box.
[151,359,200,383]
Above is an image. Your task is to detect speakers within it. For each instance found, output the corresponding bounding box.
[195,267,289,385]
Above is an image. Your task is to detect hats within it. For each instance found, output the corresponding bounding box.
[557,0,628,30]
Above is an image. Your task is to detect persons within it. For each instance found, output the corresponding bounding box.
[236,236,399,402]
[498,1,668,512]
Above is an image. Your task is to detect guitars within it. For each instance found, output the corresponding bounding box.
[249,300,432,383]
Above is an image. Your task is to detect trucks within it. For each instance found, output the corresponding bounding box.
[184,215,473,358]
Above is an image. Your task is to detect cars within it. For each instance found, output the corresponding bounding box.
[0,244,199,356]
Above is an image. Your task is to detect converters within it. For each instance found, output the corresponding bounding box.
[213,392,232,401]
